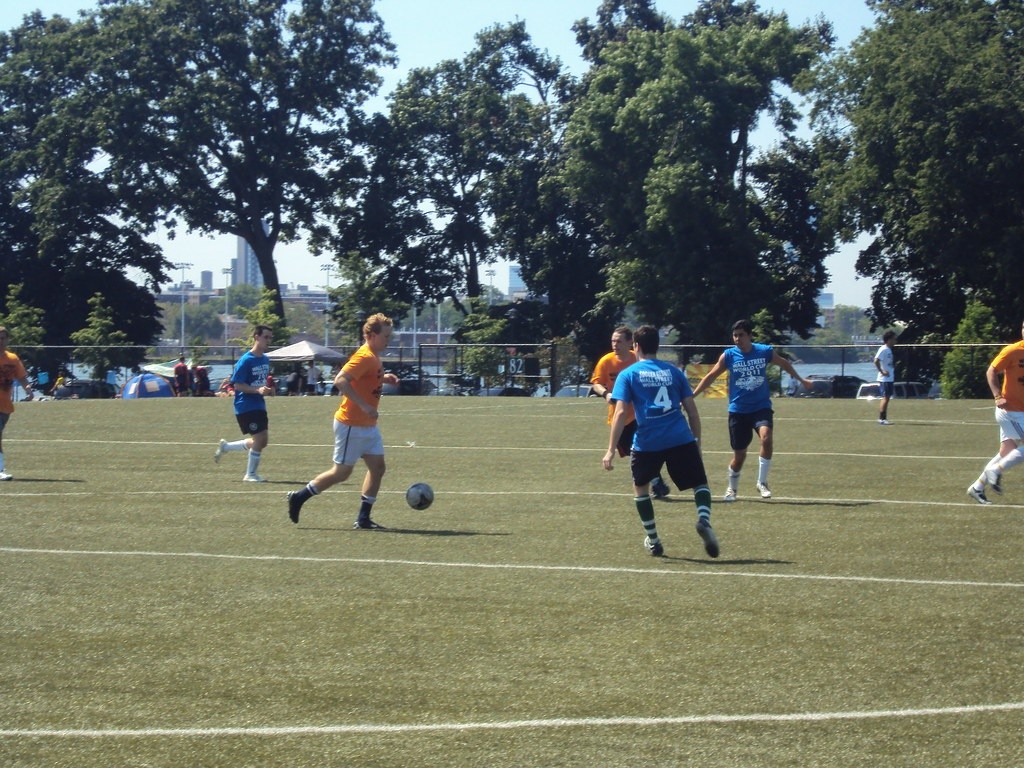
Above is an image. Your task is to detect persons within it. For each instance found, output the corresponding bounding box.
[174,357,210,397]
[681,320,813,498]
[287,313,399,530]
[306,361,322,395]
[213,325,275,482]
[602,325,720,557]
[967,321,1024,505]
[0,326,31,480]
[873,331,896,425]
[218,374,235,396]
[286,370,300,395]
[590,327,671,498]
[787,374,798,396]
[50,371,66,393]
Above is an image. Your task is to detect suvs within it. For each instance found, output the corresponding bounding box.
[794,373,869,399]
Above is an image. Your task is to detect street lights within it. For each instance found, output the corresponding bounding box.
[320,264,333,347]
[484,270,496,306]
[174,263,194,347]
[222,268,235,355]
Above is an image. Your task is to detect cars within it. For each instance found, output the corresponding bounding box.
[555,385,599,397]
[474,387,531,397]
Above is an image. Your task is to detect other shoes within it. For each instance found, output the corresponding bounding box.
[724,488,737,501]
[651,477,670,497]
[879,419,894,425]
[353,517,387,530]
[287,490,301,524]
[0,469,13,480]
[756,481,772,498]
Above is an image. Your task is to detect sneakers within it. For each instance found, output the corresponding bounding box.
[214,438,227,464]
[242,474,268,482]
[967,485,993,505]
[644,536,664,557]
[984,468,1004,496]
[695,520,720,559]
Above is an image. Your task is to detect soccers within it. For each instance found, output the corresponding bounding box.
[405,482,434,510]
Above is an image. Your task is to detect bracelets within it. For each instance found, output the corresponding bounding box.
[602,390,609,399]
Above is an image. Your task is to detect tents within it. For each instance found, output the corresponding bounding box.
[122,374,174,398]
[264,340,348,396]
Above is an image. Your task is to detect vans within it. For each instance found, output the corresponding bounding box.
[856,381,930,399]
